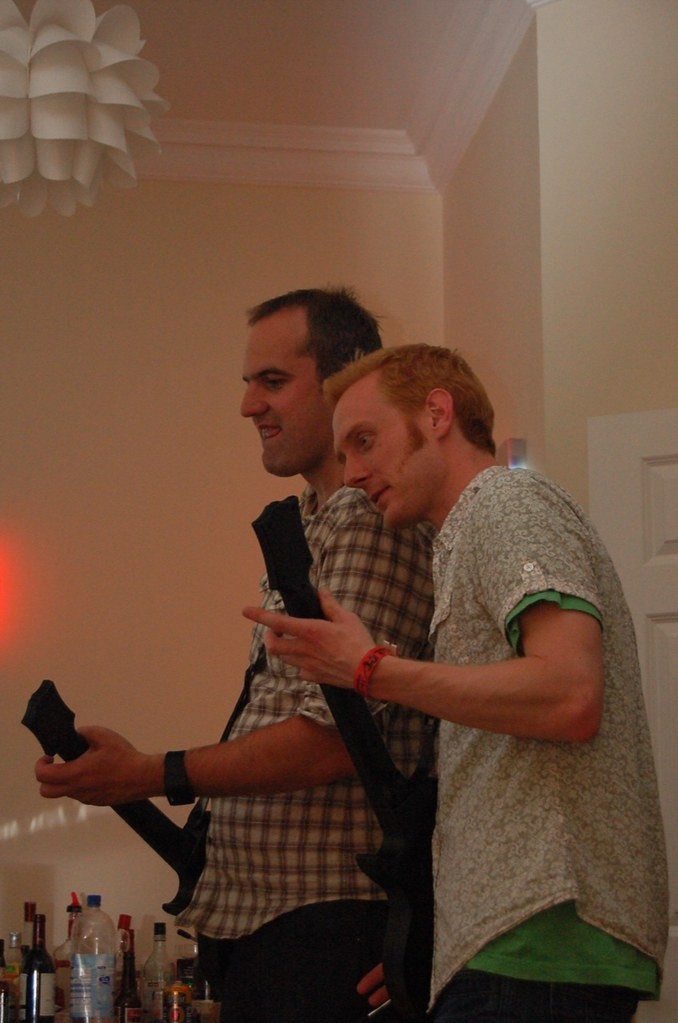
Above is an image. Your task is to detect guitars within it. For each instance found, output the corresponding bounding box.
[249,497,435,1023]
[21,675,217,919]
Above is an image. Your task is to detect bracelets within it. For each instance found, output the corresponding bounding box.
[354,639,398,703]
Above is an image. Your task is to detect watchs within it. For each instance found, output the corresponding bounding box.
[164,750,196,806]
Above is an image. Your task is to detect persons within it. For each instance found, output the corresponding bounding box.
[241,342,670,1023]
[34,290,437,1023]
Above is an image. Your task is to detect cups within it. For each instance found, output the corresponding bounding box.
[176,943,201,986]
[191,972,221,1023]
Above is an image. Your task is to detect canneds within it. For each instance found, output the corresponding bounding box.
[161,984,193,1023]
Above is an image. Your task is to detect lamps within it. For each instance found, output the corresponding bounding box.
[0,0,170,219]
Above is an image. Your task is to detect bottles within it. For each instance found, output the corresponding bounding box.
[54,892,85,1023]
[0,939,9,1023]
[140,922,177,1023]
[19,901,37,956]
[71,895,117,1023]
[5,930,32,1023]
[115,913,142,1023]
[19,914,56,1023]
